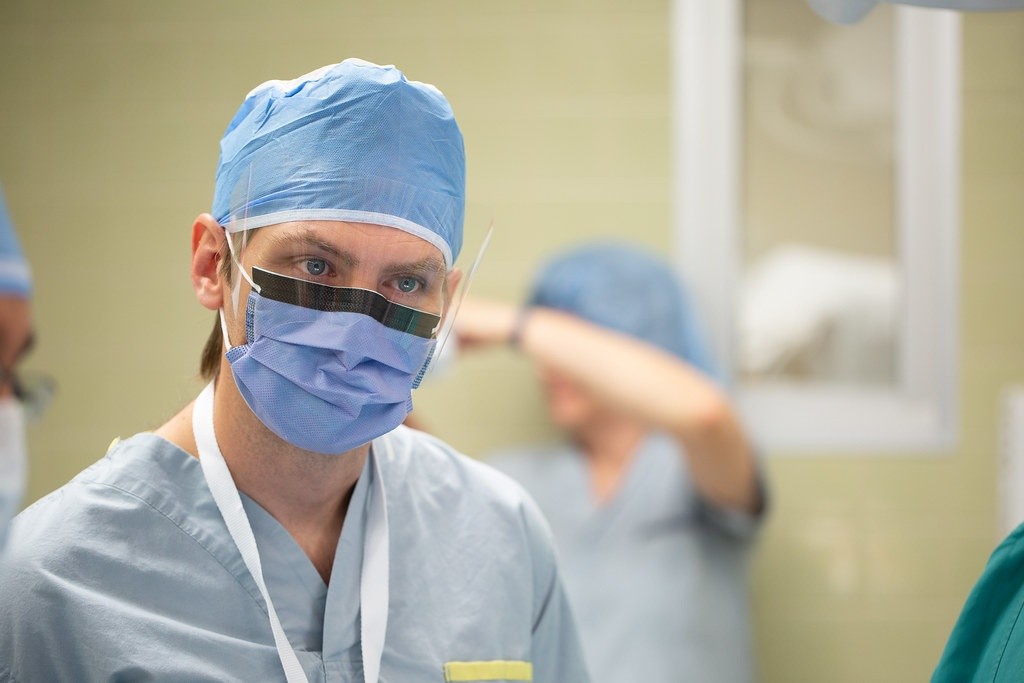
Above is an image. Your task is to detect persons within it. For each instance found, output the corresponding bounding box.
[418,235,778,682]
[1,182,46,554]
[1,54,593,683]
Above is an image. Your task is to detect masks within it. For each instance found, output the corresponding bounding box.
[219,230,441,455]
[0,400,27,555]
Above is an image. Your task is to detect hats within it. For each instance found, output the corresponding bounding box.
[532,245,714,375]
[0,205,36,299]
[208,58,465,274]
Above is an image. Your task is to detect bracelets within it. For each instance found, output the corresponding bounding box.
[508,299,535,350]
